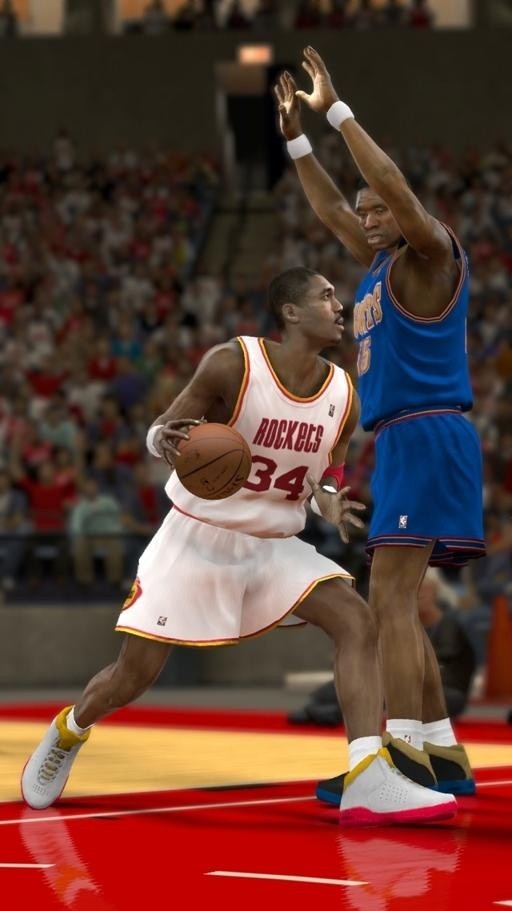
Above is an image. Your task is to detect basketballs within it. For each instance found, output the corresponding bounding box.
[176,422,252,500]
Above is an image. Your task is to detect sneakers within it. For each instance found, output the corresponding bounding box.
[20,704,90,811]
[315,729,475,826]
